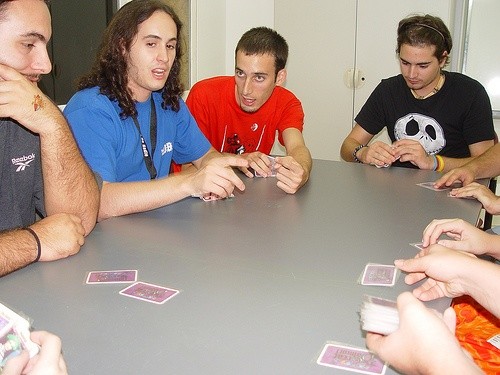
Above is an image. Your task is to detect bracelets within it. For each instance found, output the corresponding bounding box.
[19,225,41,265]
[352,144,369,164]
[431,154,445,172]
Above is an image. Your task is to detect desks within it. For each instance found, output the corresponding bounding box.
[0,154,490,375]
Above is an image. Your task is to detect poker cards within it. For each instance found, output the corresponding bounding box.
[315,340,391,375]
[409,244,424,250]
[356,295,399,336]
[254,156,282,179]
[85,269,138,285]
[1,301,43,371]
[115,281,182,305]
[356,262,401,288]
[415,181,448,192]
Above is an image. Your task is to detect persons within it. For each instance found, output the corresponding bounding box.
[170,26,313,195]
[433,140,500,235]
[62,0,249,222]
[340,13,495,171]
[3,331,68,375]
[0,0,101,278]
[423,217,500,261]
[365,243,500,375]
